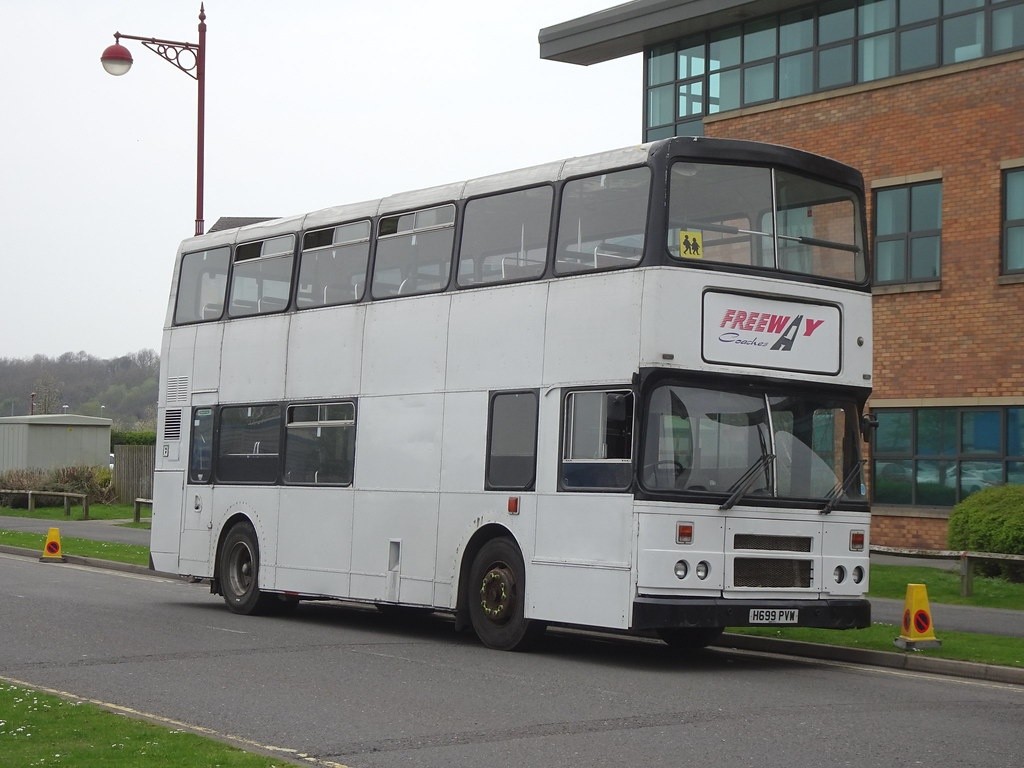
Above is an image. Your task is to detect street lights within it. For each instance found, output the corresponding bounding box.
[101,2,206,237]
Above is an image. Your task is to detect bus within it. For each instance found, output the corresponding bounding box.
[148,136,872,650]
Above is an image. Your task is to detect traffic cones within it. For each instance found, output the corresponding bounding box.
[39,527,66,562]
[893,583,943,653]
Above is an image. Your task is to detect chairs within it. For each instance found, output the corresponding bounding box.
[202,244,643,320]
[314,468,336,483]
